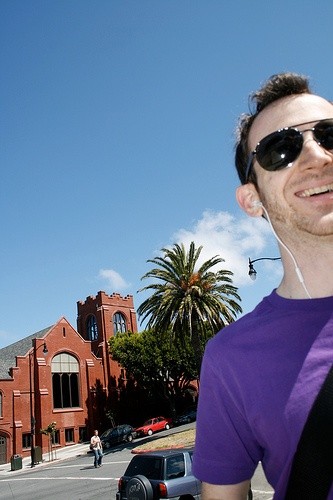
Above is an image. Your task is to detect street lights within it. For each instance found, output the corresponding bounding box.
[28,343,48,467]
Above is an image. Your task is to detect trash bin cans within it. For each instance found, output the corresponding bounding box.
[10,454,22,471]
[31,446,42,465]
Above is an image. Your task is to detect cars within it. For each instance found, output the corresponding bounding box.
[135,416,174,438]
[90,424,136,451]
[175,410,196,427]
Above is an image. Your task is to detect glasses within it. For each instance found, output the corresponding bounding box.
[244,119,333,185]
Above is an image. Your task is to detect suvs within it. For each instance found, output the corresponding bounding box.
[116,447,253,500]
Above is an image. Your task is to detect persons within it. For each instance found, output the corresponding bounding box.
[192,70,333,500]
[91,430,103,467]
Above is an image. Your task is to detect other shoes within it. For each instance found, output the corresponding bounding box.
[95,465,102,468]
